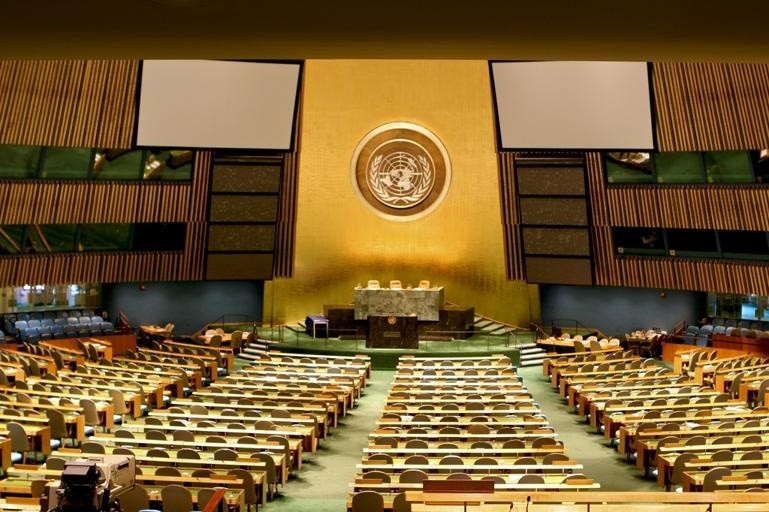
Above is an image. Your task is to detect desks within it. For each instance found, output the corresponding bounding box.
[355,287,445,322]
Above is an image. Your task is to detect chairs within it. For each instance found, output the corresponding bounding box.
[368,279,430,287]
